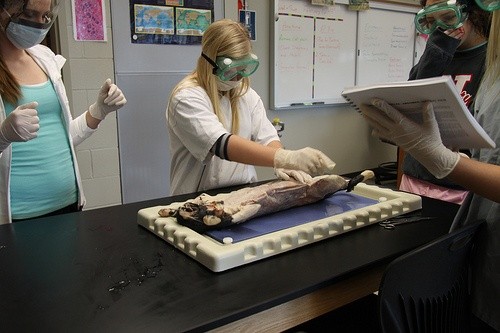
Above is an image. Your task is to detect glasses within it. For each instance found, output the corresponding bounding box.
[475,0,500,11]
[201,52,259,82]
[414,0,479,35]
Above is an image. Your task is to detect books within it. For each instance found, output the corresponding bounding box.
[341,76,497,148]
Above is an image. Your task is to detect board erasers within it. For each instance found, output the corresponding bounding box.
[291,103,304,105]
[312,102,324,106]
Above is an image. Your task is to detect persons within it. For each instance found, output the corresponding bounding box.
[407,0,492,164]
[165,20,336,197]
[0,0,127,227]
[361,0,500,235]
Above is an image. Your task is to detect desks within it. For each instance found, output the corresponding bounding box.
[0,166,461,333]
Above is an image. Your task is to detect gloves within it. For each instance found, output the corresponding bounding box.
[88,79,127,121]
[0,102,40,152]
[273,147,337,185]
[358,98,460,179]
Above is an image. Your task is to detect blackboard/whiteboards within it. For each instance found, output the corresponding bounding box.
[271,0,419,112]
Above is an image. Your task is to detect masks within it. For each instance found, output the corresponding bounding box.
[428,22,464,54]
[216,77,245,91]
[0,5,54,50]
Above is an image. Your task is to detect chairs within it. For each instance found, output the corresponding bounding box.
[303,218,488,333]
